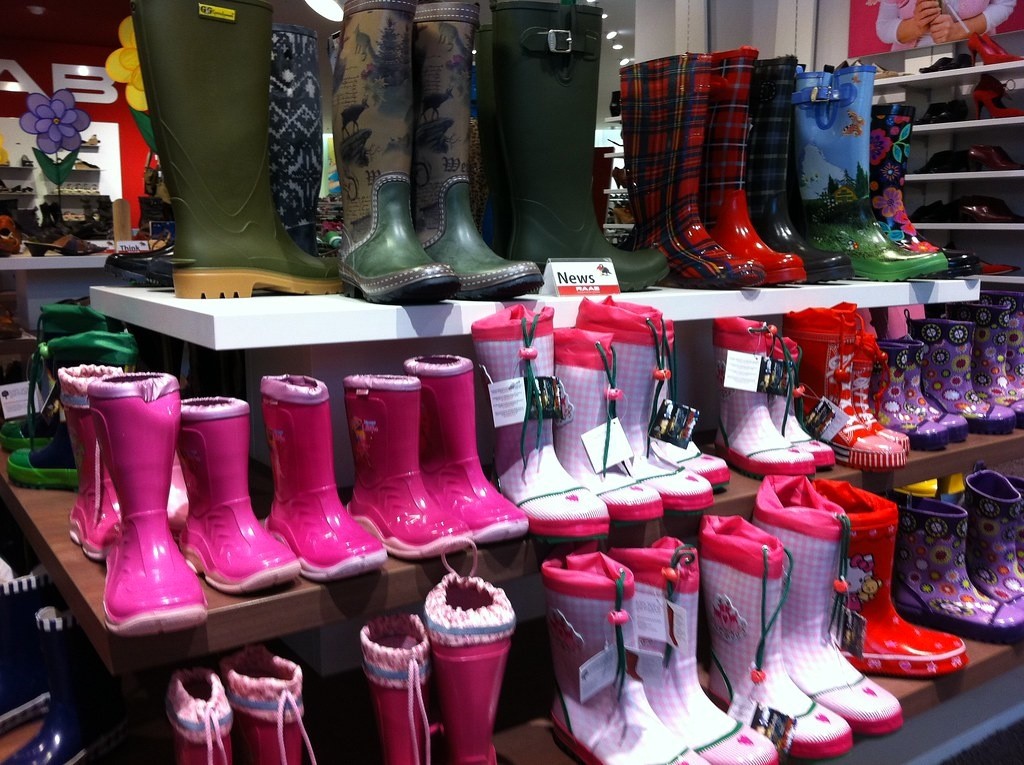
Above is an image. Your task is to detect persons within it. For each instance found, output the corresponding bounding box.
[875,0,1017,51]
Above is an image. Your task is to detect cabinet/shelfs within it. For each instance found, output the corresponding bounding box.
[873,60,1024,285]
[0,279,1024,765]
[603,116,636,229]
[0,144,103,221]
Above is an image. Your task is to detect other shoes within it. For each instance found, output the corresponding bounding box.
[0,134,114,419]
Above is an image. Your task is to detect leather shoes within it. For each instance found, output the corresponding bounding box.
[822,33,1024,275]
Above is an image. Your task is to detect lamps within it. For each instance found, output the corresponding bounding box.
[305,0,346,23]
[587,0,630,66]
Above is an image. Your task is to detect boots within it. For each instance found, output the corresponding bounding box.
[0,302,1024,765]
[100,0,985,305]
